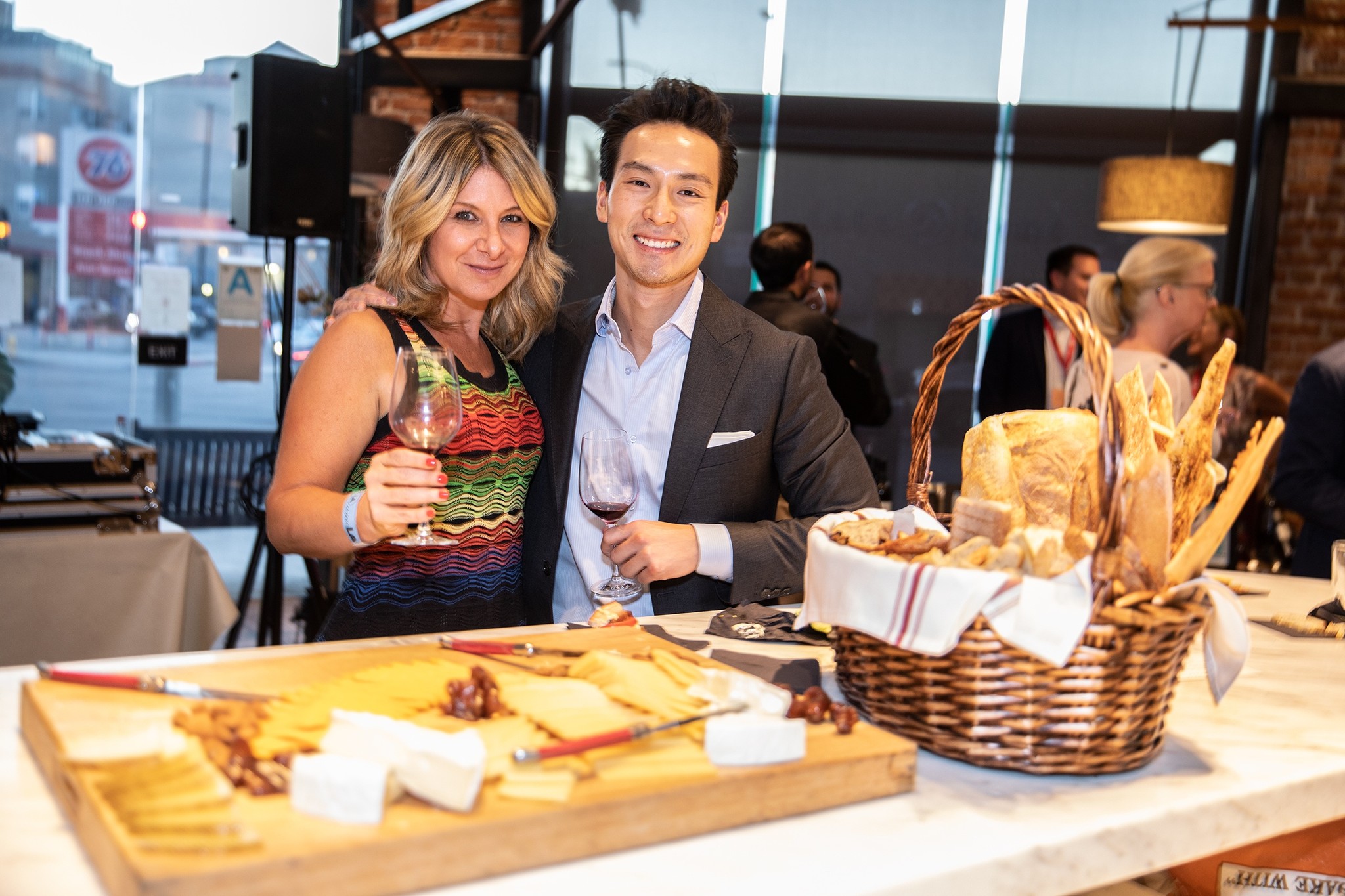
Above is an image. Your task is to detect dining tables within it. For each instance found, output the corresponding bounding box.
[0,570,1345,896]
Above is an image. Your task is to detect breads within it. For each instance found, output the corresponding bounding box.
[39,652,716,860]
[832,338,1285,599]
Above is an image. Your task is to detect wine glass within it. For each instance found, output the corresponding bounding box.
[577,429,643,597]
[377,344,465,549]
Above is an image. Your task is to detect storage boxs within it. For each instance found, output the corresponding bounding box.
[0,430,160,537]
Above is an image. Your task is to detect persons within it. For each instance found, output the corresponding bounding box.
[807,261,877,363]
[1182,303,1290,478]
[264,106,577,626]
[319,77,882,623]
[1268,341,1345,578]
[977,245,1102,423]
[1061,236,1224,455]
[739,222,889,431]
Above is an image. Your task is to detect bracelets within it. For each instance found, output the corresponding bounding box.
[342,489,373,549]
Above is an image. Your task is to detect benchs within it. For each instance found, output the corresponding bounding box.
[132,420,278,527]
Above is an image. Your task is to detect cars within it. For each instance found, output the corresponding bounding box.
[37,287,327,365]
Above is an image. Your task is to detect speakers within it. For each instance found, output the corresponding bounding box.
[229,52,349,240]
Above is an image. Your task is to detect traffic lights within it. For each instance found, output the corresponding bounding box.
[0,207,9,244]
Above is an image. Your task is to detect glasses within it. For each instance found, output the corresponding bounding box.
[1156,281,1216,298]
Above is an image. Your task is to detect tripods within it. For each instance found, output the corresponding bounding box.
[219,243,333,652]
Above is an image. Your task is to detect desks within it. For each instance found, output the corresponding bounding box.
[1,514,240,668]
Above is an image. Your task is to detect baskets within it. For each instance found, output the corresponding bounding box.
[790,279,1248,777]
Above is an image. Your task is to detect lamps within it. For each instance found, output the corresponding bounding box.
[1098,21,1234,234]
